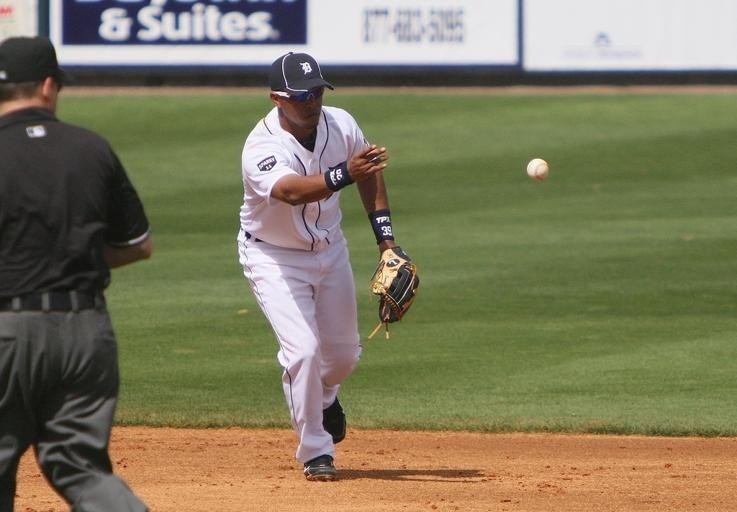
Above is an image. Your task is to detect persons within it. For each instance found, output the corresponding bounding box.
[235,52,418,483]
[0,34,155,511]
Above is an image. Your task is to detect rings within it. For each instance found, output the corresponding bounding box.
[368,155,381,165]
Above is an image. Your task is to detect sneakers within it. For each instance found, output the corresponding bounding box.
[303,453,337,481]
[322,396,347,443]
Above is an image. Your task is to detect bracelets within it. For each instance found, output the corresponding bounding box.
[323,158,354,193]
[366,208,395,246]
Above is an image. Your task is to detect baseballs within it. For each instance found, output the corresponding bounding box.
[527,158,550,182]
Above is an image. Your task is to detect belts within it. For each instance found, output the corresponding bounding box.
[0,289,106,313]
[240,226,264,243]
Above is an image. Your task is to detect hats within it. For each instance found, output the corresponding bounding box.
[0,37,66,89]
[268,49,336,99]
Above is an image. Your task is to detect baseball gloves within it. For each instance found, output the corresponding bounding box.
[371,242,420,323]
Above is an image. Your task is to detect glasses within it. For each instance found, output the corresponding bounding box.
[294,88,325,101]
